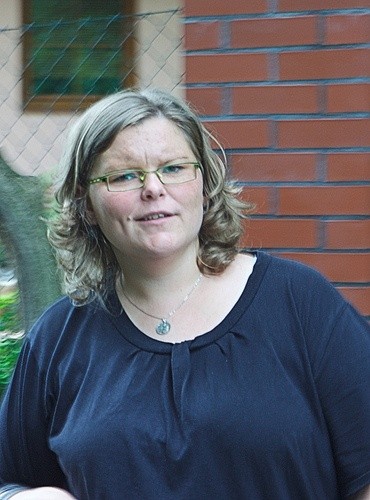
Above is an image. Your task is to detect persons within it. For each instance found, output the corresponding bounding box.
[0,88,370,500]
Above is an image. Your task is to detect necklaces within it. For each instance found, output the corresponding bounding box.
[120,261,205,336]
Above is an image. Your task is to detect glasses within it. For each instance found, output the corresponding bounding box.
[85,161,204,193]
